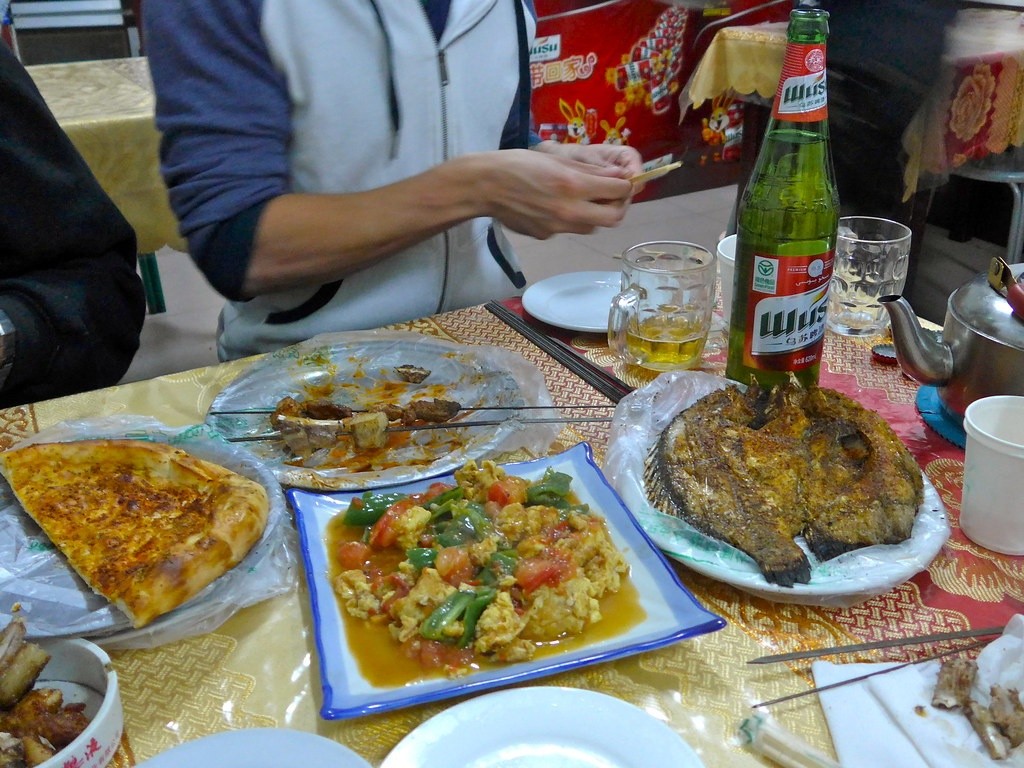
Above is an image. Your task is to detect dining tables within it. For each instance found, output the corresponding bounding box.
[0,257,1024,768]
[21,53,200,310]
[674,8,1024,305]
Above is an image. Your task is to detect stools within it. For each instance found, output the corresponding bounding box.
[937,153,1024,271]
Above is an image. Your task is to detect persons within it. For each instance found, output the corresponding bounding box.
[138,0,645,364]
[1,38,145,409]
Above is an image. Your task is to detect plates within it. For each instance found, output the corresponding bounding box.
[204,337,525,492]
[604,374,950,599]
[375,685,706,767]
[523,271,691,331]
[282,439,729,724]
[0,426,283,641]
[121,727,374,768]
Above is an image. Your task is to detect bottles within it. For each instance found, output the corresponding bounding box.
[724,7,840,389]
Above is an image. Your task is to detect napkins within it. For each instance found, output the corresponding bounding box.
[812,612,1024,768]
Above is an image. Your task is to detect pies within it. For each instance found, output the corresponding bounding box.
[0,439,268,628]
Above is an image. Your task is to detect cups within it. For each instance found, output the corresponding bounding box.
[717,233,741,327]
[960,392,1023,557]
[607,241,716,374]
[827,215,913,337]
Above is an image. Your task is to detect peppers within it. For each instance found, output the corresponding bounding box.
[342,470,590,646]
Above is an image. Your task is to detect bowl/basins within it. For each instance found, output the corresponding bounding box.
[0,637,124,768]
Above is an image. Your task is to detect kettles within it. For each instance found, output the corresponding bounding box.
[876,252,1024,434]
